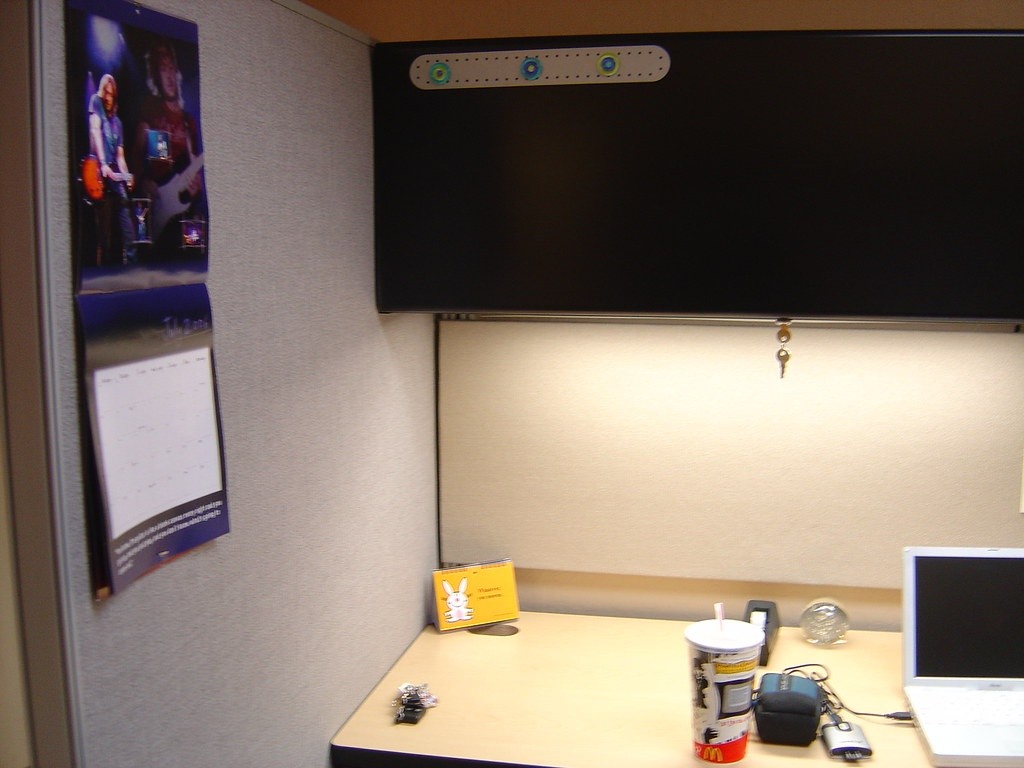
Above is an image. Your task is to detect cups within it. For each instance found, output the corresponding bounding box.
[684,618,765,764]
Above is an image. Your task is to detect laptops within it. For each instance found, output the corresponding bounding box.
[903,548,1024,768]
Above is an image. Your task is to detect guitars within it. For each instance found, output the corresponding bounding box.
[145,150,204,241]
[78,153,133,206]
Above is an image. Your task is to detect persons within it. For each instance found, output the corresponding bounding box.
[86,72,142,280]
[132,39,210,271]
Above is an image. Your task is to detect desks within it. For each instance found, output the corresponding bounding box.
[331,612,937,768]
